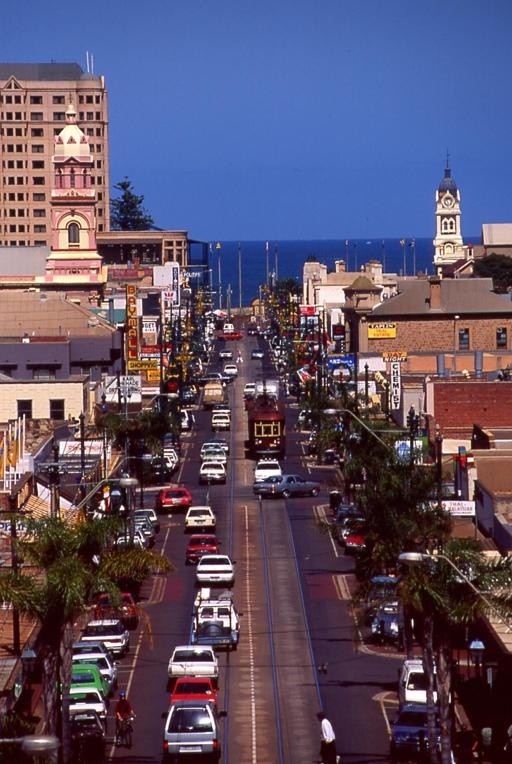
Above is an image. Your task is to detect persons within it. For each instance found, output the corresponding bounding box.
[315,710,343,764]
[114,689,137,746]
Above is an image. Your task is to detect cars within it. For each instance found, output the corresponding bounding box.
[332,490,418,641]
[71,653,123,688]
[114,312,236,562]
[218,330,245,343]
[70,640,110,655]
[398,658,454,705]
[387,702,441,754]
[196,552,237,586]
[249,473,321,498]
[68,662,113,705]
[246,327,259,336]
[251,456,283,483]
[250,347,266,359]
[168,675,219,705]
[243,381,255,397]
[166,644,220,680]
[68,688,110,723]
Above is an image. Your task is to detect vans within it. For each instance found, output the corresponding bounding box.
[297,408,313,426]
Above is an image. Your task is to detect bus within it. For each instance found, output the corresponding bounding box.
[242,393,288,462]
[255,379,280,401]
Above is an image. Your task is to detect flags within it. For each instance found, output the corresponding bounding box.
[207,235,416,256]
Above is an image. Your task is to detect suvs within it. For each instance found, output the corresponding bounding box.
[93,590,140,630]
[158,700,229,762]
[79,619,133,658]
[188,586,242,651]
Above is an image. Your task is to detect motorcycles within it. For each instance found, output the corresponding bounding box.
[112,714,136,751]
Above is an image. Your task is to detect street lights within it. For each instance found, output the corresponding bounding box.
[319,406,401,460]
[318,307,330,392]
[315,310,323,408]
[395,549,511,634]
[406,406,421,468]
[451,313,459,372]
[468,636,485,681]
[47,477,139,532]
[21,640,38,719]
[0,731,64,754]
